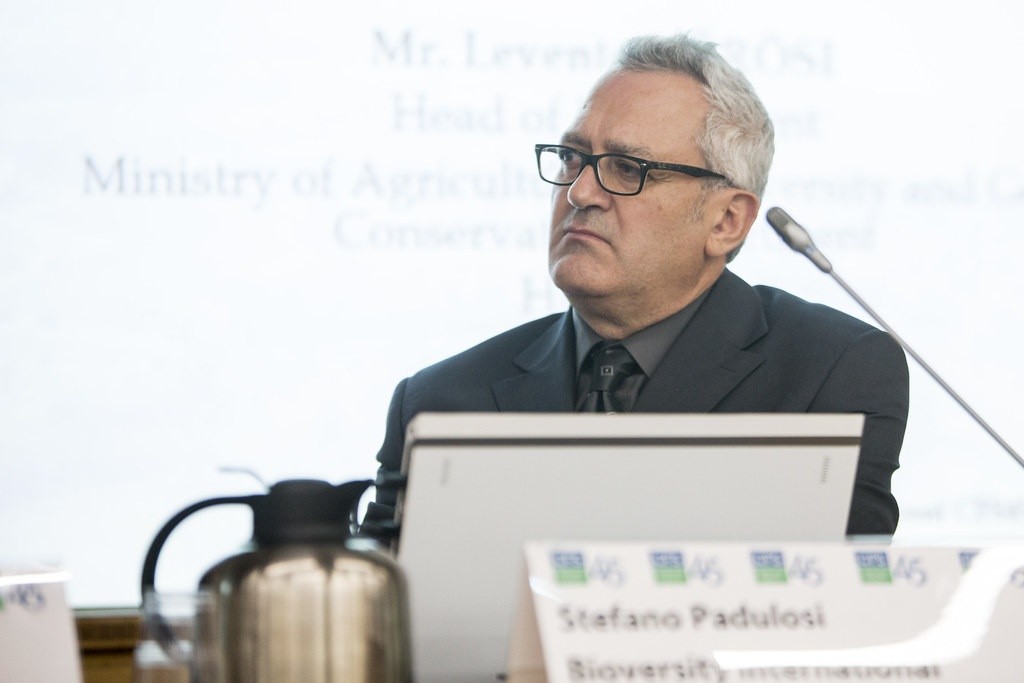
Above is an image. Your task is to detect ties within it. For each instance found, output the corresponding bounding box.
[579,342,638,412]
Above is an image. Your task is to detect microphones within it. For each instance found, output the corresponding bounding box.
[766,207,1024,468]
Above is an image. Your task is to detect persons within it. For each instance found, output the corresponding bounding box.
[358,30,910,541]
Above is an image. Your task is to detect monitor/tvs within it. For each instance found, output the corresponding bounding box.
[395,412,864,683]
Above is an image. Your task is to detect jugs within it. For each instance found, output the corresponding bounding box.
[140,477,413,683]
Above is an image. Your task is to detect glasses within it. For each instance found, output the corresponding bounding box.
[535,144,736,196]
